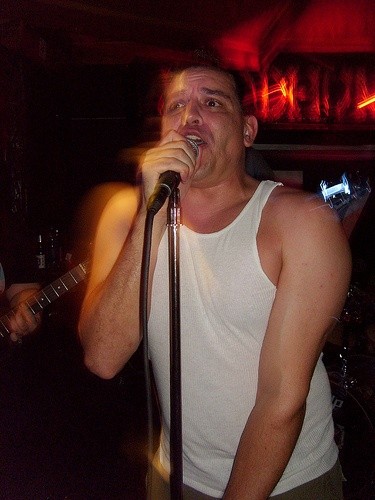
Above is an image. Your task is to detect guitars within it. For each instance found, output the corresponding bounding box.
[0,259,92,341]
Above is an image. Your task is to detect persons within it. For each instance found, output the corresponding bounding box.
[0,214,43,347]
[78,54,352,500]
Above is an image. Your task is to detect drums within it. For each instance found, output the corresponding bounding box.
[327,371,375,495]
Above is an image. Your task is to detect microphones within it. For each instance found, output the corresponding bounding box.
[147,138,199,216]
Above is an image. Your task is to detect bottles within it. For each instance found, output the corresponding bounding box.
[35,226,67,272]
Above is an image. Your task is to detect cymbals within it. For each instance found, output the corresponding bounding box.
[326,285,375,350]
